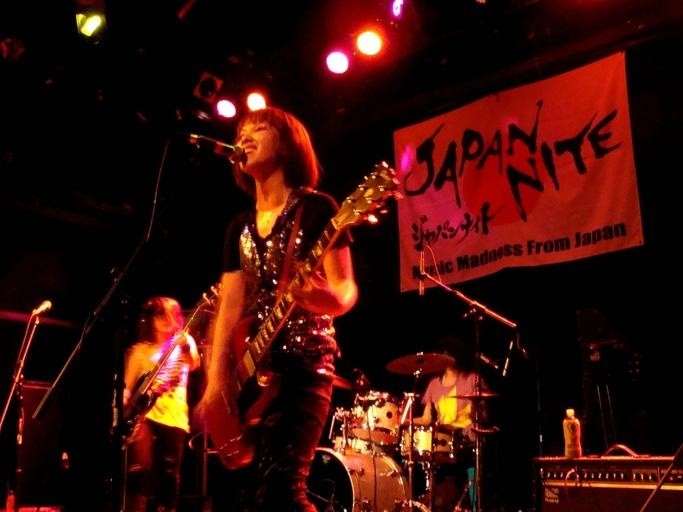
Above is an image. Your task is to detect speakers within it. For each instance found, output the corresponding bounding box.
[14,379,61,505]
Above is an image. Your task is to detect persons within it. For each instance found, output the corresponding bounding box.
[403,346,493,510]
[119,297,200,511]
[191,108,359,512]
[471,333,522,393]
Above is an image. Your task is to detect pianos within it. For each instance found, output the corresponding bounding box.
[533,454,683,492]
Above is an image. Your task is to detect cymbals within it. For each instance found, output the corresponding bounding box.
[332,374,353,391]
[447,390,501,401]
[0,310,71,328]
[387,352,455,374]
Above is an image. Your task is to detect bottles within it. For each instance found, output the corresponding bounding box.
[561,408,583,458]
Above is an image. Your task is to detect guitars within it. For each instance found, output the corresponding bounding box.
[120,283,220,441]
[205,163,400,469]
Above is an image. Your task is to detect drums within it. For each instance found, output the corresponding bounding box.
[350,391,403,446]
[396,420,461,466]
[305,446,407,512]
[16,381,51,475]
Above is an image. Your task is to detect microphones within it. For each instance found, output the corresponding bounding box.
[33,301,52,316]
[188,133,243,164]
[417,250,426,296]
